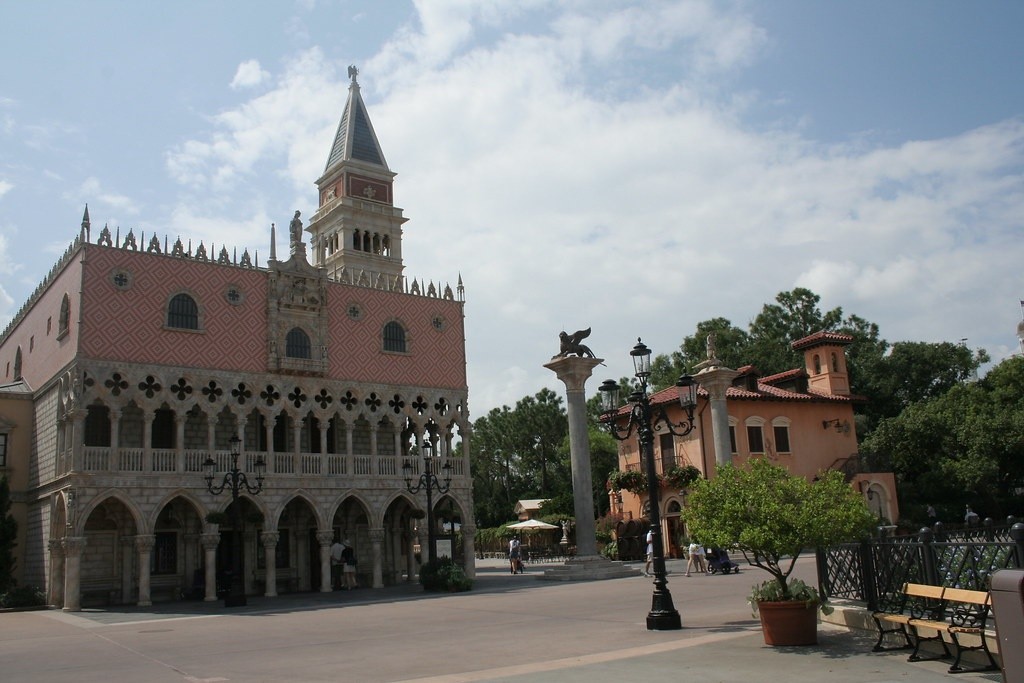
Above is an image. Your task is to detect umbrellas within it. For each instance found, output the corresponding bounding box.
[506,519,559,556]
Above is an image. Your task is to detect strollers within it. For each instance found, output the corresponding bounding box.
[703,544,739,575]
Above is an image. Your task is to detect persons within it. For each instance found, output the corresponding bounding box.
[289,210,303,243]
[331,538,359,591]
[966,503,972,514]
[561,520,569,537]
[644,530,653,577]
[926,502,936,528]
[707,331,717,360]
[509,536,521,574]
[684,543,710,577]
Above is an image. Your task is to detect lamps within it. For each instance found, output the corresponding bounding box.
[823,418,843,433]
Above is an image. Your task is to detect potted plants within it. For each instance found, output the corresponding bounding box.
[678,449,878,647]
[436,554,465,592]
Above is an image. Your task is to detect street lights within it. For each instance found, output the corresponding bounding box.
[402,441,455,572]
[203,433,268,608]
[597,336,699,630]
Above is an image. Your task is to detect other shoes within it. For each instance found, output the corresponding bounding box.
[706,572,712,576]
[685,573,690,576]
[644,572,652,577]
[514,572,518,574]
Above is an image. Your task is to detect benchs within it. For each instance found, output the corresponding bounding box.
[867,581,1003,674]
